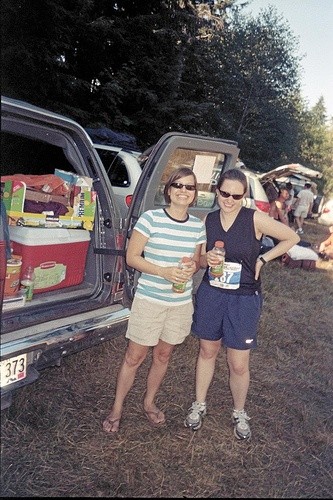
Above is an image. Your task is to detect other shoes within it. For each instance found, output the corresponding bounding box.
[296,228,304,234]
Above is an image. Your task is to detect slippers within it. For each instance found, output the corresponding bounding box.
[144,408,167,426]
[102,416,121,435]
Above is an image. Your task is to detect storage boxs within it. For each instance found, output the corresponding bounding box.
[0,225,90,292]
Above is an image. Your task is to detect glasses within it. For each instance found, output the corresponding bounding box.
[217,187,246,200]
[170,182,196,191]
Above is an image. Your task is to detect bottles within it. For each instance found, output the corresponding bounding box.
[210,241,226,277]
[20,266,36,302]
[172,257,191,294]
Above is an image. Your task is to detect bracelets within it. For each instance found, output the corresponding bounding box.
[259,255,267,264]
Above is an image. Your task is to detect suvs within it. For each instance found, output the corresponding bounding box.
[176,161,323,223]
[93,142,158,224]
[0,96,241,425]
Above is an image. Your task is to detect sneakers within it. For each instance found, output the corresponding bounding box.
[231,410,252,442]
[183,402,207,431]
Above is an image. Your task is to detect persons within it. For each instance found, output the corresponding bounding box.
[271,189,293,226]
[285,182,314,235]
[184,170,301,441]
[102,167,207,434]
